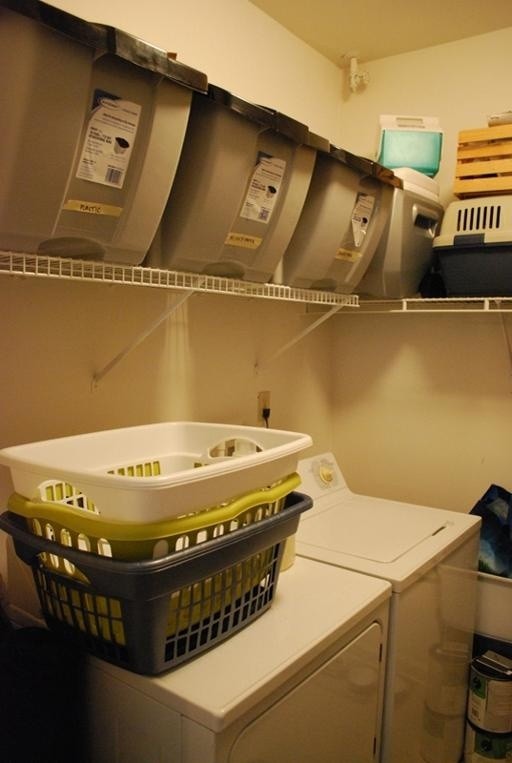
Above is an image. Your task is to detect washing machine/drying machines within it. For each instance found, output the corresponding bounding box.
[269,453,482,762]
[6,534,394,762]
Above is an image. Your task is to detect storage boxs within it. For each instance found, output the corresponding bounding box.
[351,167,445,300]
[269,144,403,294]
[0,0,208,265]
[142,82,331,283]
[474,569,512,645]
[431,195,512,297]
